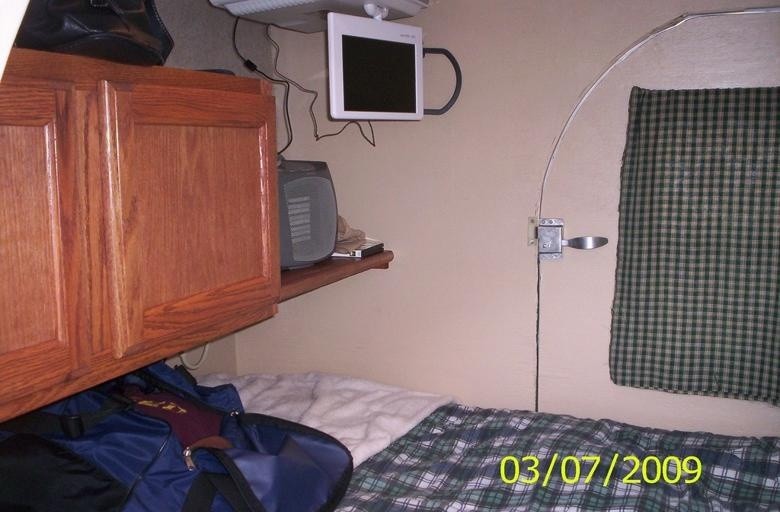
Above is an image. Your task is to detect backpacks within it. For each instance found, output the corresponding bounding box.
[0,361,355,512]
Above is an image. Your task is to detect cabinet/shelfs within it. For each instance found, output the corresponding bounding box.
[0,46,282,424]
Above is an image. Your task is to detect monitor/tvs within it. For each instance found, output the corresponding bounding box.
[326,12,424,121]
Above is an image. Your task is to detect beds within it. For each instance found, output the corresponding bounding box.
[195,374,780,511]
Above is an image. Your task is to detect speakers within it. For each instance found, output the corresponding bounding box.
[278,159,339,268]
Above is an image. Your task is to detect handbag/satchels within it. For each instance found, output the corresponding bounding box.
[13,0,175,67]
